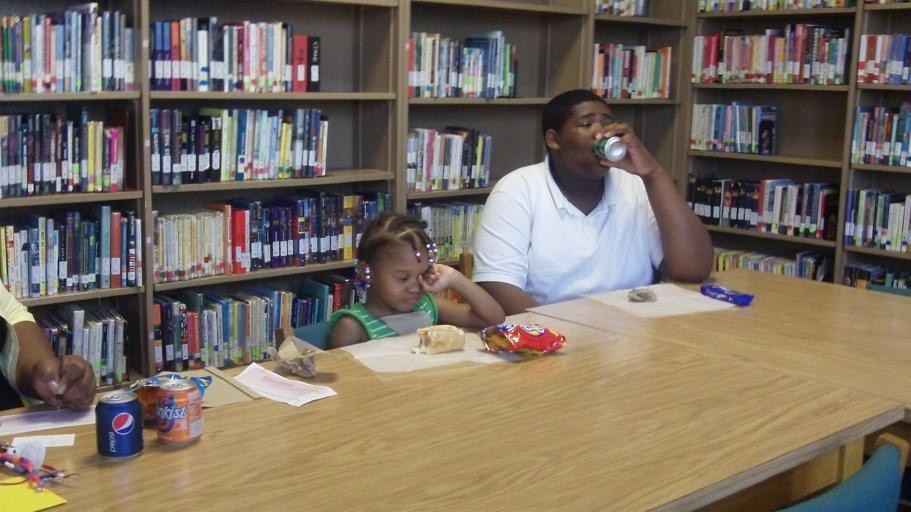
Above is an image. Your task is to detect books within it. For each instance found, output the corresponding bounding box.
[590,0,674,99]
[407,29,518,304]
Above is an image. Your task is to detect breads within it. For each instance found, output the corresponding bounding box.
[415,325,465,353]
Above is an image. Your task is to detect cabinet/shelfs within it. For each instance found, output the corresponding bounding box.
[682,1,909,290]
[0,0,695,410]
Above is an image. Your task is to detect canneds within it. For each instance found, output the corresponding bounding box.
[95,391,144,458]
[592,136,627,163]
[156,380,202,444]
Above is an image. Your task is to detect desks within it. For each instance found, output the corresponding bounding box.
[525,268,911,475]
[0,310,905,510]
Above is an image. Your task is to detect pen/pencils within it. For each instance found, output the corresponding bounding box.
[55,329,68,411]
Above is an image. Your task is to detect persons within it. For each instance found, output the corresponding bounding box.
[0,280,98,412]
[326,214,507,351]
[471,88,714,312]
